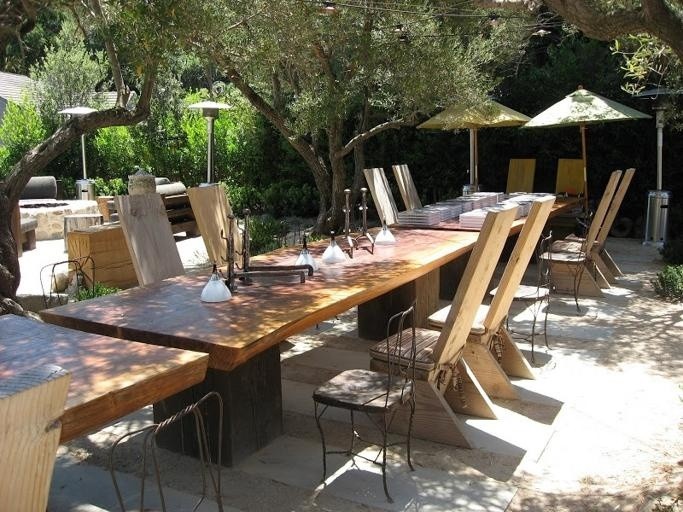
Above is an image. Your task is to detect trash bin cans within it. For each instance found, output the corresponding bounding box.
[642,191,670,248]
[75,179,95,200]
[462,184,488,196]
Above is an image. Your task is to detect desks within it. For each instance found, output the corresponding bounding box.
[39,227,478,469]
[1,314,209,441]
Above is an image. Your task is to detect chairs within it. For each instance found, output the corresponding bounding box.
[106,390,224,511]
[311,299,418,503]
[489,231,554,362]
[1,362,71,511]
[360,157,635,314]
[426,195,557,400]
[16,169,242,312]
[369,203,518,452]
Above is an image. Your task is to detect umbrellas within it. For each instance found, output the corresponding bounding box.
[416,94,533,190]
[516,81,655,224]
